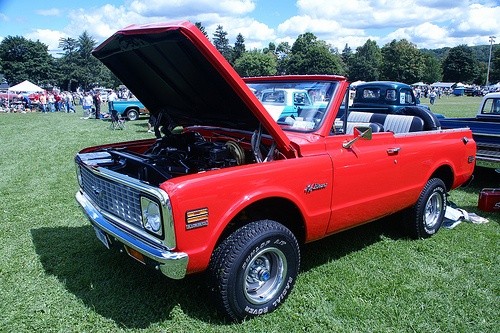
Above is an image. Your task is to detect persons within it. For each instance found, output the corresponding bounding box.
[148,114,163,133]
[413,86,453,105]
[466,84,499,96]
[0,83,135,120]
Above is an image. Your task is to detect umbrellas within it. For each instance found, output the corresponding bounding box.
[456,82,465,86]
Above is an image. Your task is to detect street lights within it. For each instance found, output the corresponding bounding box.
[486,35,496,86]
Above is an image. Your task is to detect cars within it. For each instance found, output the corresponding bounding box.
[464,82,500,97]
[90,84,135,102]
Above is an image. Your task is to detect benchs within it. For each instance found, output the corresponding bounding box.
[341,111,424,133]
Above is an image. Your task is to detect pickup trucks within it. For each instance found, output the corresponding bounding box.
[107,99,150,121]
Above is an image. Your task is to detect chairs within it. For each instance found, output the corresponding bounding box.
[346,121,383,135]
[111,110,127,131]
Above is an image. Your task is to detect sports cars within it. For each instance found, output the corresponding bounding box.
[250,81,445,125]
[438,91,500,162]
[75,19,478,324]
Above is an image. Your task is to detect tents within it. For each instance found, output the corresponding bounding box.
[351,80,366,86]
[8,80,45,108]
[412,81,443,87]
